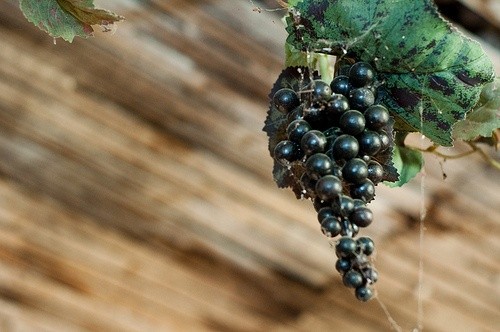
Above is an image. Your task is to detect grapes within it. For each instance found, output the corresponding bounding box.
[272,62,390,301]
